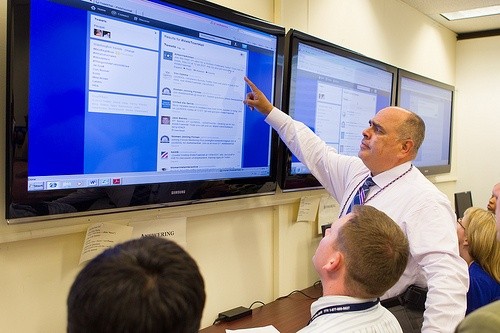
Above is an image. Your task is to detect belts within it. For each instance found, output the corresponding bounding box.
[380,296,405,309]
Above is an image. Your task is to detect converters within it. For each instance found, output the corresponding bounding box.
[219,305,254,322]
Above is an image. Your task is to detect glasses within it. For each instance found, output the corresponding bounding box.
[457,217,467,232]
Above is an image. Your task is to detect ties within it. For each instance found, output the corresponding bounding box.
[346,177,376,213]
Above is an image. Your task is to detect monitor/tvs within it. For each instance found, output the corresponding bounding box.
[5,0,287,219]
[281,28,399,191]
[394,67,455,176]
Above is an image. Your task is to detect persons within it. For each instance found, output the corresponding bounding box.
[456,207,500,316]
[486,184,500,213]
[67,238,207,333]
[243,72,470,332]
[295,204,411,333]
[454,184,500,333]
